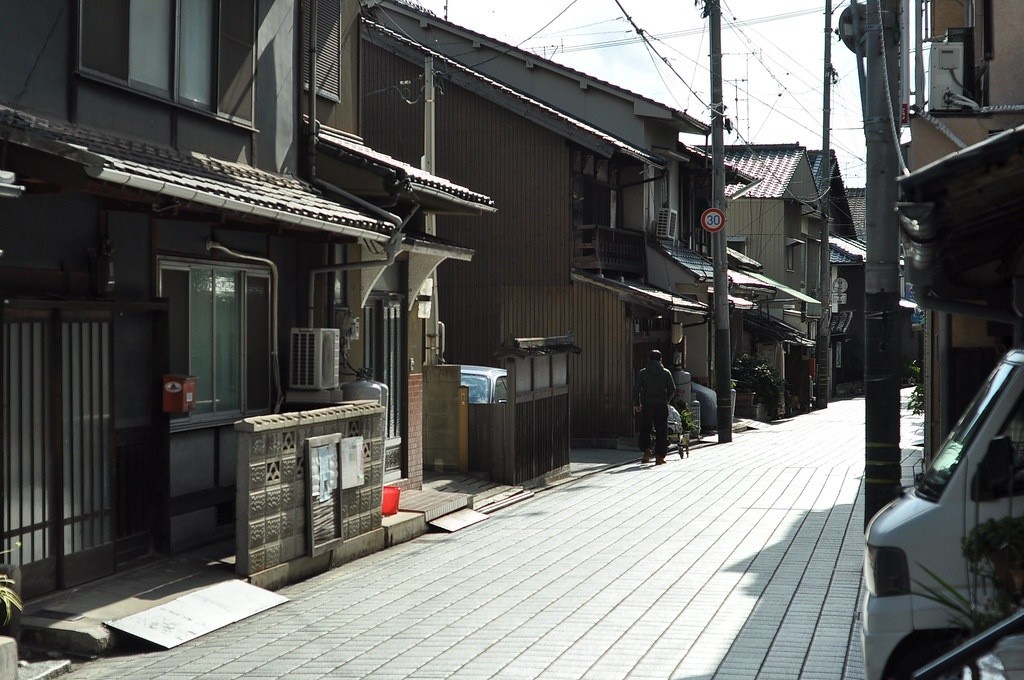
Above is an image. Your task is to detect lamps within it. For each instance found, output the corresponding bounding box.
[414,278,434,319]
[671,322,683,344]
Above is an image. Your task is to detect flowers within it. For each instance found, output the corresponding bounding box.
[730,352,777,397]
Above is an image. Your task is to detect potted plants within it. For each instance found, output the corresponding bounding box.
[960,515,1024,601]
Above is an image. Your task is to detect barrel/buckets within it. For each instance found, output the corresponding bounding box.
[382,486,402,515]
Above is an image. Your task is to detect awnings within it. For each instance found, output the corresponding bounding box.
[743,271,822,305]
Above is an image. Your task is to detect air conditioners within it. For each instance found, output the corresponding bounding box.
[656,208,678,240]
[803,302,822,319]
[289,327,339,391]
[928,41,964,111]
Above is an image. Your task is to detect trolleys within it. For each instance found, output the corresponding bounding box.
[644,420,693,458]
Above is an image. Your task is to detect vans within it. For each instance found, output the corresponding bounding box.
[859,348,1024,680]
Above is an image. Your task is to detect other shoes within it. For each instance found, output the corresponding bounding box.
[642,448,653,464]
[655,457,666,464]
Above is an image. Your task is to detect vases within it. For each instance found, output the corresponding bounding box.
[753,397,764,404]
[735,392,756,406]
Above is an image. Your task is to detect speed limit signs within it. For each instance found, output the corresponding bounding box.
[700,209,725,232]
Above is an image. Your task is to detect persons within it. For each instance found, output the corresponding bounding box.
[636,349,678,464]
